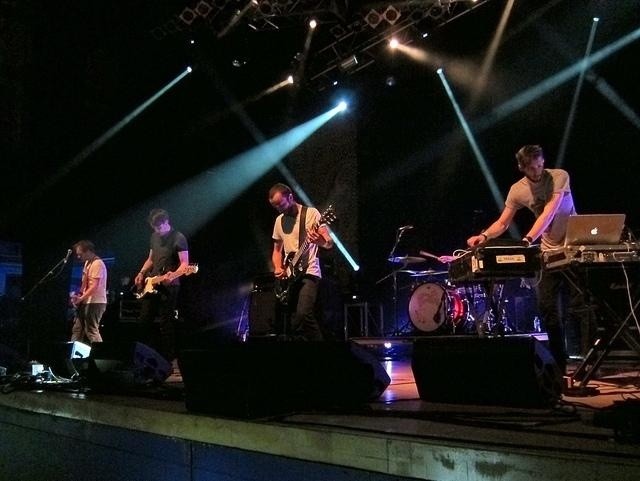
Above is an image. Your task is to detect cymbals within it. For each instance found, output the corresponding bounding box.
[390,256,424,264]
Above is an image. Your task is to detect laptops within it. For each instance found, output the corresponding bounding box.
[561,214,626,245]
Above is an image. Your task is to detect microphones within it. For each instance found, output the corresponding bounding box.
[398,225,414,231]
[64,249,72,263]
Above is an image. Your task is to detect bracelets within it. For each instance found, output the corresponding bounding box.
[524,236,532,242]
[481,234,487,241]
[139,272,145,278]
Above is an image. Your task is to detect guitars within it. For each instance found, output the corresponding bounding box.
[132,264,198,299]
[274,205,336,305]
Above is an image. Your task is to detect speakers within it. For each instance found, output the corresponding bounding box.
[410,333,562,407]
[176,347,303,416]
[248,335,390,411]
[89,342,173,389]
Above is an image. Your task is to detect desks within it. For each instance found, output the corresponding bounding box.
[344,298,384,339]
[547,260,640,395]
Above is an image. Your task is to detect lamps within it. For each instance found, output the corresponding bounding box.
[164,1,487,100]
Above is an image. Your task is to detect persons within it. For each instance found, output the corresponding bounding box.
[269,183,333,341]
[70,240,107,342]
[134,209,189,383]
[467,145,601,377]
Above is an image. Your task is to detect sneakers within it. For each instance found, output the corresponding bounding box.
[576,365,601,381]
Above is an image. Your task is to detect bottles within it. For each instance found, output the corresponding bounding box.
[534,317,541,332]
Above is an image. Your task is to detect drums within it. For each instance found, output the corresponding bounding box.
[409,270,451,293]
[407,280,470,334]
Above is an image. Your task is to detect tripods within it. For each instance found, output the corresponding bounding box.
[381,254,409,337]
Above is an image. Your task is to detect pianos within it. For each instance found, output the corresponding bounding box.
[450,246,541,284]
[545,243,640,269]
